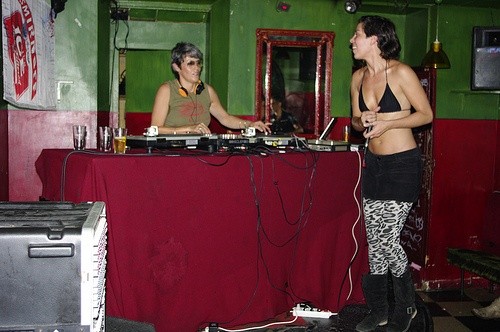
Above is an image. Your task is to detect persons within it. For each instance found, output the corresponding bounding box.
[349,15,433,332]
[270,95,303,134]
[151,43,270,134]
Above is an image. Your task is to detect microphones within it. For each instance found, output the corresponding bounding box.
[363,125,372,156]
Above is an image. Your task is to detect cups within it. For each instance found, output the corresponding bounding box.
[72,125,86,151]
[112,128,128,155]
[343,125,352,143]
[98,126,112,152]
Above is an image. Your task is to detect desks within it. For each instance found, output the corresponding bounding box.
[35,149,367,332]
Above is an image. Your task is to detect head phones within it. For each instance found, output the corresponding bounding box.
[176,79,205,98]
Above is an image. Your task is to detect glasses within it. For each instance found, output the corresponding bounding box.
[180,60,203,68]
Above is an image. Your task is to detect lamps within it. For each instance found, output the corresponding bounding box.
[344,0,361,15]
[419,0,451,70]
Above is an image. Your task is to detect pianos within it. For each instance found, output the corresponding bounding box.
[124,131,309,152]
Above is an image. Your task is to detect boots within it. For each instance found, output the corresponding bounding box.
[355,273,389,332]
[374,271,423,332]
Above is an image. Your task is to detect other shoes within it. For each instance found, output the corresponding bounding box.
[471,298,500,319]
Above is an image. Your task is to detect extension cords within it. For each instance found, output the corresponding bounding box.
[292,308,332,319]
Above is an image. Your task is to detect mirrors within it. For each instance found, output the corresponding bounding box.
[254,27,335,138]
